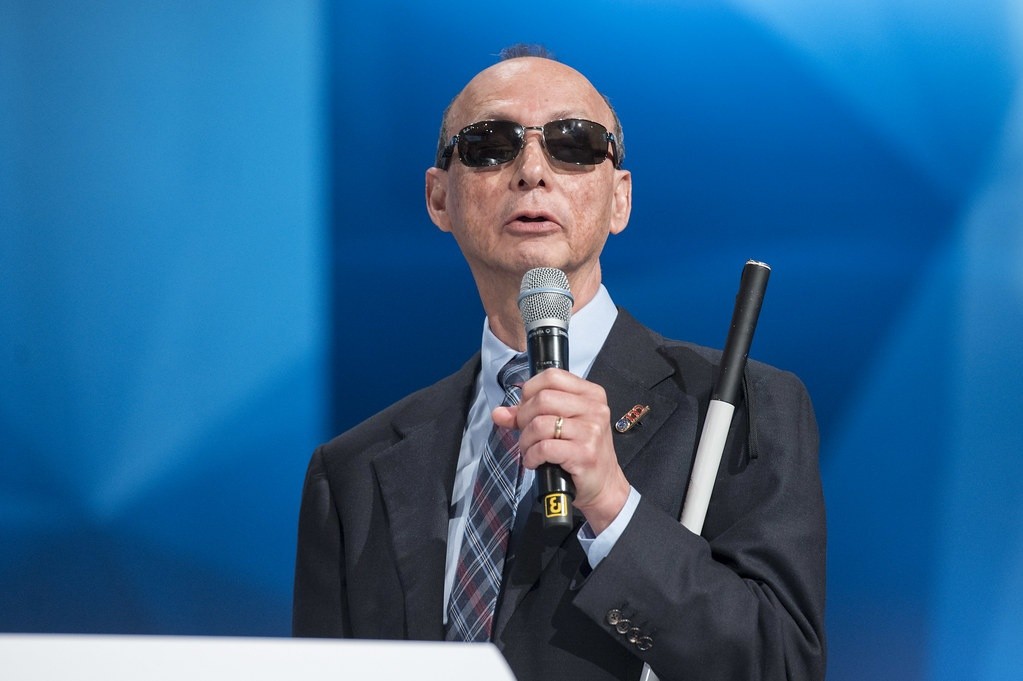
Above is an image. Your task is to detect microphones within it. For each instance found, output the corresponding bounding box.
[515,267,576,537]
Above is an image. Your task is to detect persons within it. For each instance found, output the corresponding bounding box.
[293,47,827,681]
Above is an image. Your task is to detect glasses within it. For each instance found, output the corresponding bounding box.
[440,118,619,172]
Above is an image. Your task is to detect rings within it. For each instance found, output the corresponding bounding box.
[555,416,562,439]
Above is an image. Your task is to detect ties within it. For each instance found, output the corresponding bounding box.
[444,354,533,643]
